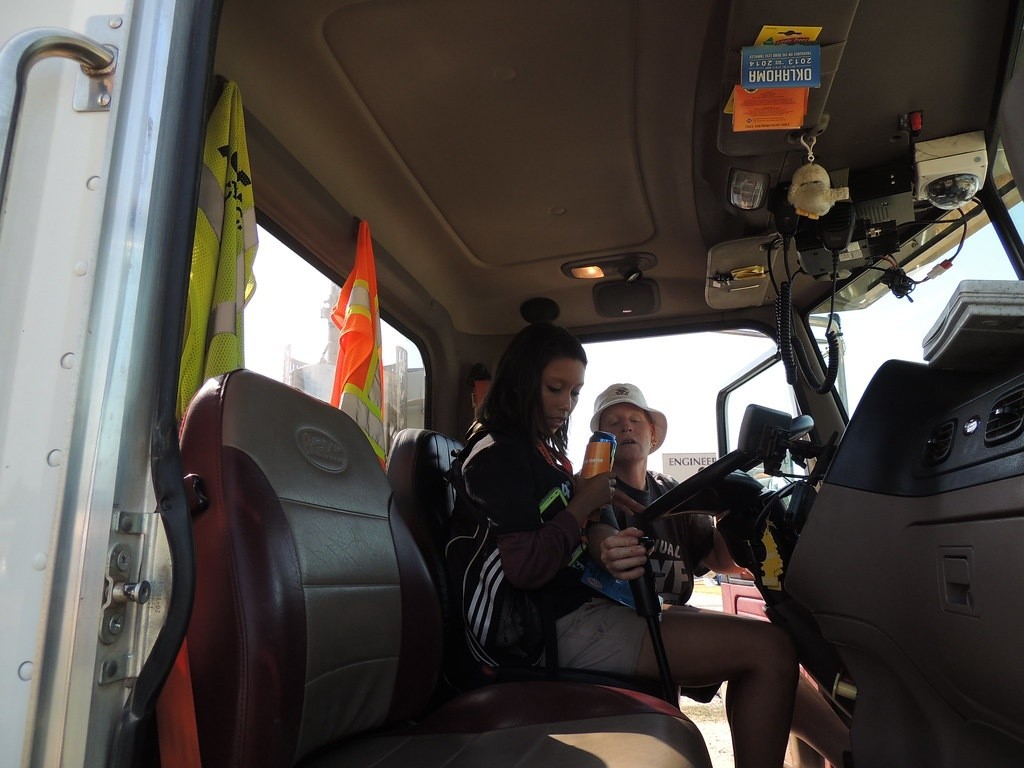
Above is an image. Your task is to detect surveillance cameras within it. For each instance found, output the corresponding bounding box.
[915,150,988,210]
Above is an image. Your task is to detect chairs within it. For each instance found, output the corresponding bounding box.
[186,366,715,768]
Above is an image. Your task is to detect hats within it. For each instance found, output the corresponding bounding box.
[591,384,667,454]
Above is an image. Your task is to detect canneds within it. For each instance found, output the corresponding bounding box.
[581,430,617,478]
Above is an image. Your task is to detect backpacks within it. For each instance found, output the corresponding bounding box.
[435,464,546,670]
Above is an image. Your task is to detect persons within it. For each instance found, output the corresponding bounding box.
[580,384,749,611]
[441,296,850,767]
